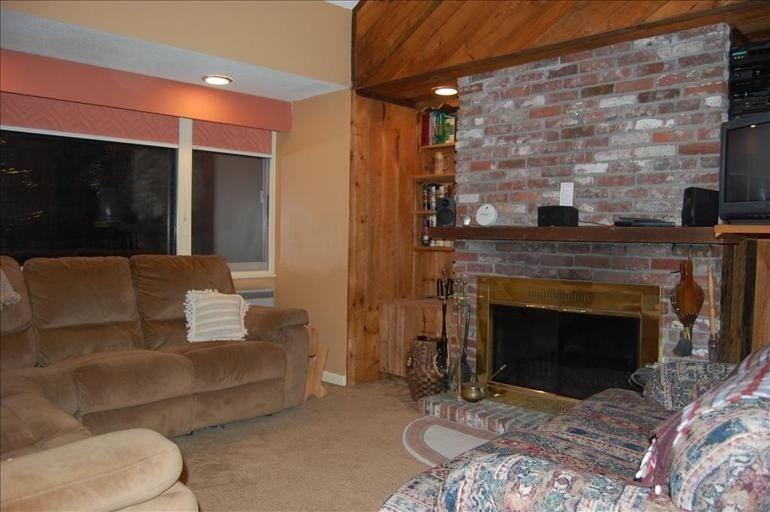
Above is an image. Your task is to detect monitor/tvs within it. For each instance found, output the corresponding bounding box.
[718,112,769,225]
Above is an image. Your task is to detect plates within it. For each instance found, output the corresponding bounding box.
[476,204,497,226]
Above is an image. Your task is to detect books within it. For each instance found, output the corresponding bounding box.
[421,181,455,247]
[421,111,456,145]
[614,219,675,226]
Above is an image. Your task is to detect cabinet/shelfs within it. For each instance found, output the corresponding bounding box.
[413,101,458,252]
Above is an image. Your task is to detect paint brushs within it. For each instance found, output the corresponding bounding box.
[707,265,720,362]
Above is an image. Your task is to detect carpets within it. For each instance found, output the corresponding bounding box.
[401,415,498,467]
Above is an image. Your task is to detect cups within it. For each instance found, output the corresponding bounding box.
[462,216,472,226]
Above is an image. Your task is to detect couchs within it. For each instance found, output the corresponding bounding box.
[380,344,769,511]
[1,254,308,512]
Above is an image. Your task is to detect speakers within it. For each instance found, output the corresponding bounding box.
[437,198,455,227]
[682,188,717,228]
[538,206,579,228]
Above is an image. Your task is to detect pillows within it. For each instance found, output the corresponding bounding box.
[183,289,248,343]
